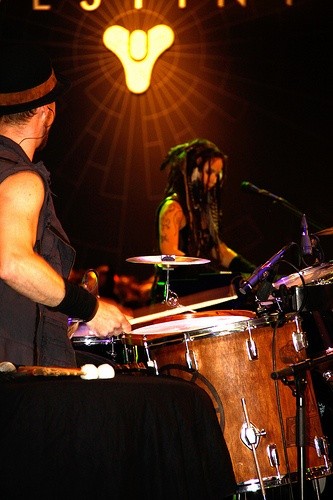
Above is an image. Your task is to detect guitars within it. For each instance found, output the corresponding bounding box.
[231,241,295,311]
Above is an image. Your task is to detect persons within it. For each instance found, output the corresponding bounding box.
[157,139,257,298]
[0,22,132,367]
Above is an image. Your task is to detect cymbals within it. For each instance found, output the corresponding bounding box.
[126,255,212,265]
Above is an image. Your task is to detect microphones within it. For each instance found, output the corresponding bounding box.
[300,215,313,256]
[238,242,292,294]
[261,189,290,204]
[242,181,269,198]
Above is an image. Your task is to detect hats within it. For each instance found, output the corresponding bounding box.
[1,55,71,115]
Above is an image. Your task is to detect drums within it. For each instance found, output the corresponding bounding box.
[273,257,333,447]
[145,311,333,492]
[70,309,256,374]
